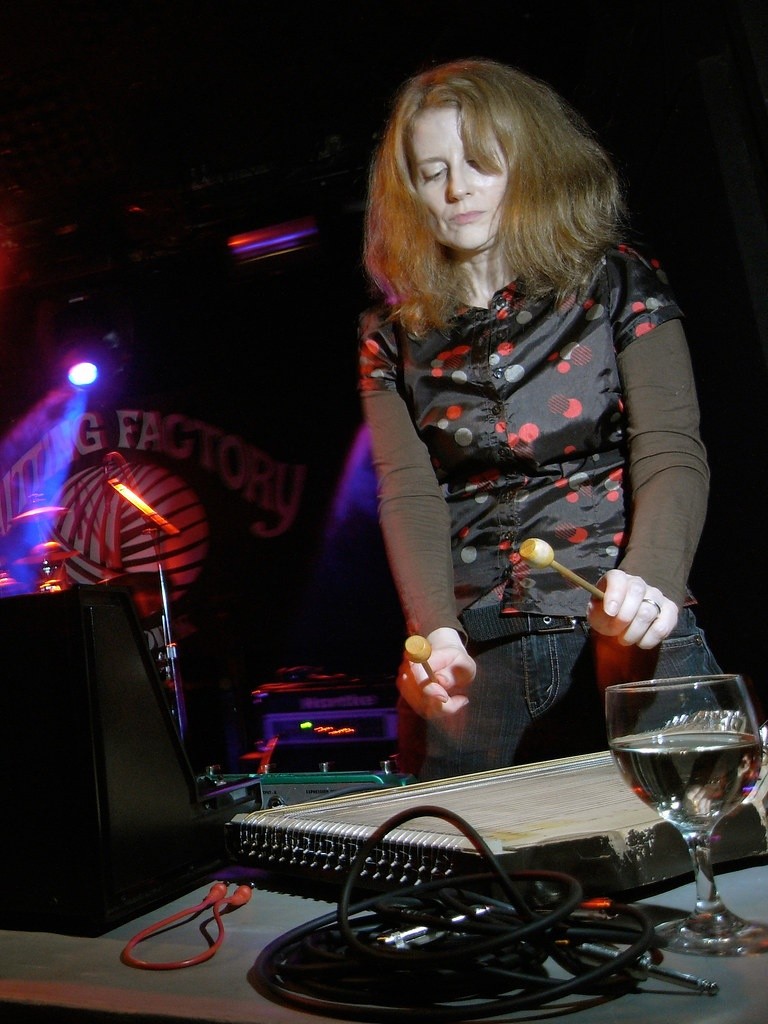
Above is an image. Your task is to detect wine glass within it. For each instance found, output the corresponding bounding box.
[605,675,768,958]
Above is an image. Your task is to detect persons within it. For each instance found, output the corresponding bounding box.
[356,60,724,779]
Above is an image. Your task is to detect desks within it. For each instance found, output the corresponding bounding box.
[0,782,767,1023]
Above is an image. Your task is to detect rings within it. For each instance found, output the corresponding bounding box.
[642,598,662,616]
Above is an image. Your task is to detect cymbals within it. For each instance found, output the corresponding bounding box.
[14,550,81,564]
[13,506,69,524]
